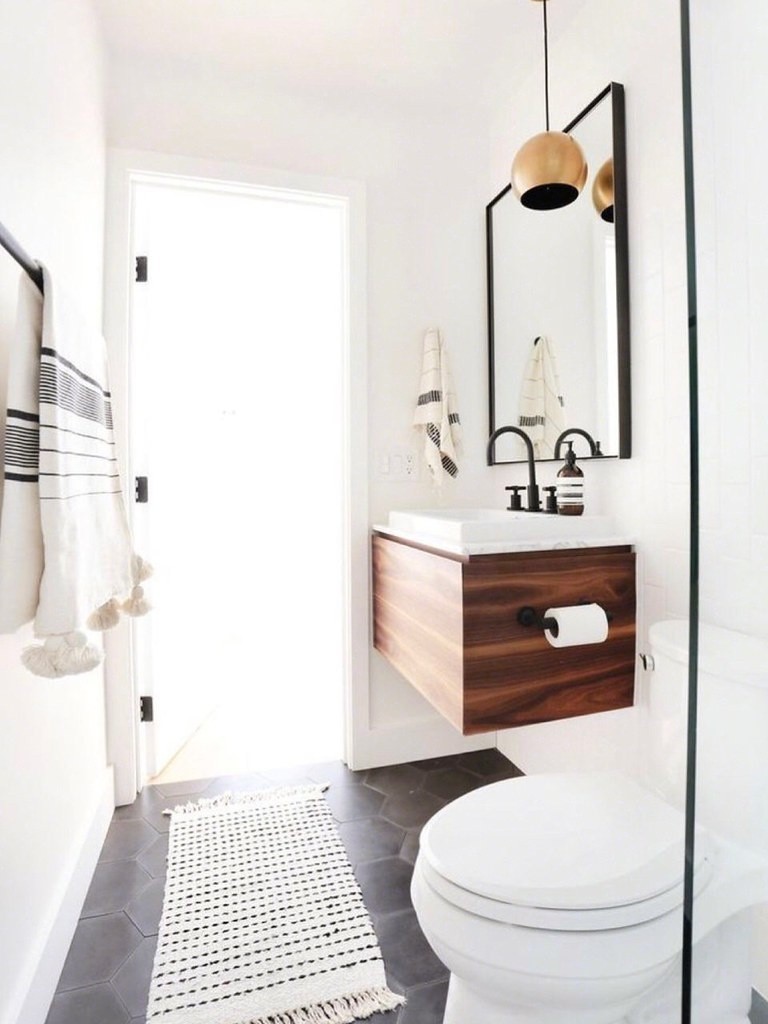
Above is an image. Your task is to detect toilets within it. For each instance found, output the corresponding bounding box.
[409,614,768,1024]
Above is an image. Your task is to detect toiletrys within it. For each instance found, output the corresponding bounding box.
[554,438,587,516]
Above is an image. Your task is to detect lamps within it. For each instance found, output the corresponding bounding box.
[509,0,591,211]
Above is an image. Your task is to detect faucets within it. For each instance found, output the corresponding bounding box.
[486,424,542,511]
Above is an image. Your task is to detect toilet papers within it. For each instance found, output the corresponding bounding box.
[544,602,609,648]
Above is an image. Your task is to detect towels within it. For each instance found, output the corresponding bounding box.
[514,330,567,460]
[414,326,472,490]
[0,257,156,682]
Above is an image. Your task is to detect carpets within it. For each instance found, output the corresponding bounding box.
[147,779,411,1023]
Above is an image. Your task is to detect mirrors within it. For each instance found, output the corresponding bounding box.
[485,82,633,466]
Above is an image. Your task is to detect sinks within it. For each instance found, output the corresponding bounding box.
[385,499,618,552]
